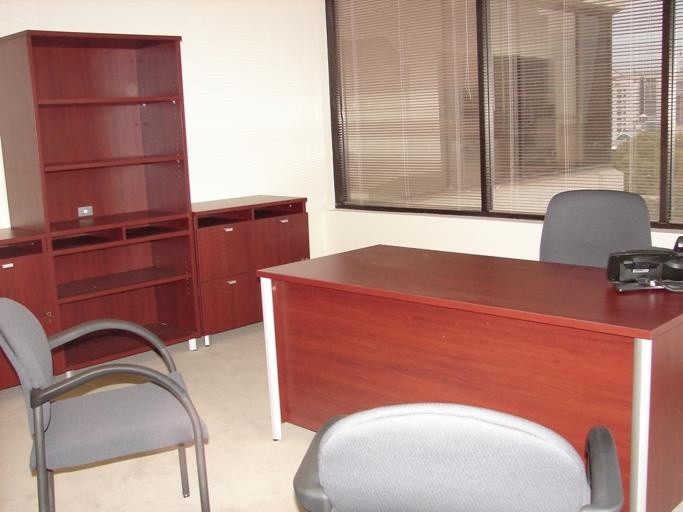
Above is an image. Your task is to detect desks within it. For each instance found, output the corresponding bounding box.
[253,244,683,512]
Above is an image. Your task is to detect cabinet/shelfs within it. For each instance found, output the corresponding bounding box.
[0,29,200,392]
[191,194,310,348]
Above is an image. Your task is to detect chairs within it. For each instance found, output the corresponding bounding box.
[293,400,623,512]
[538,189,653,268]
[0,297,212,512]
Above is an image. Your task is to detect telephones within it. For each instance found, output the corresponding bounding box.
[607,236,683,292]
